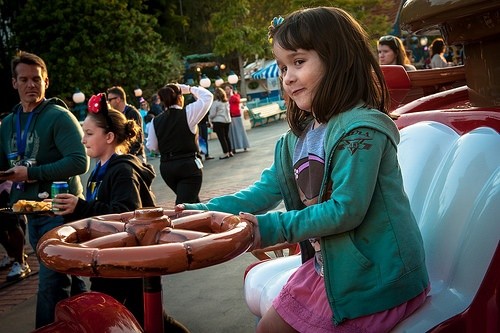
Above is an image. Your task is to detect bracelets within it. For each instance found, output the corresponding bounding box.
[189,86,191,93]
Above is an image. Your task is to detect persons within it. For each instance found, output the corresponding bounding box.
[43,93,190,333]
[175,7,431,333]
[0,52,90,330]
[226,85,250,159]
[107,86,162,176]
[378,35,416,71]
[146,83,213,205]
[430,38,464,67]
[208,88,234,159]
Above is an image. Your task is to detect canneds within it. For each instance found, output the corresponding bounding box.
[24,158,37,183]
[7,152,21,168]
[51,181,69,213]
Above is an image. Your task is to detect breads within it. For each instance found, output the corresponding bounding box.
[13,200,52,212]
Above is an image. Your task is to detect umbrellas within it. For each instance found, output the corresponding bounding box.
[251,59,282,100]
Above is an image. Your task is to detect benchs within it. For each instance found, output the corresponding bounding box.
[243,110,500,333]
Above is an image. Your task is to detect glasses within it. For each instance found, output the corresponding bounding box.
[108,97,117,101]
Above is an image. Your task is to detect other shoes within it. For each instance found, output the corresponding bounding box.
[0,255,14,266]
[220,155,229,159]
[230,153,234,156]
[206,157,214,160]
[8,262,31,278]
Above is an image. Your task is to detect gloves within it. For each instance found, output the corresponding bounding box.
[177,84,190,94]
